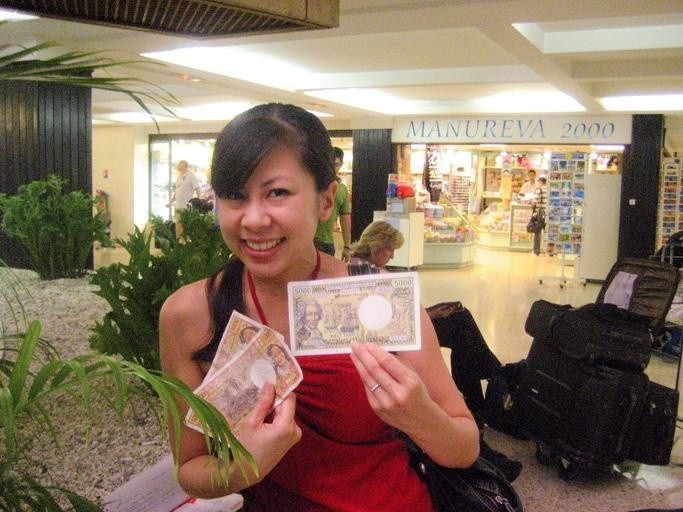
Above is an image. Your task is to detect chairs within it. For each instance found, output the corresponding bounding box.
[594,255,681,322]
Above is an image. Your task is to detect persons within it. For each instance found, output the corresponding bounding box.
[160,103,478,510]
[313,147,352,263]
[347,221,526,427]
[519,169,554,257]
[170,160,200,244]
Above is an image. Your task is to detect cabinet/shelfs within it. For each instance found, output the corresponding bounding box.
[372,211,425,272]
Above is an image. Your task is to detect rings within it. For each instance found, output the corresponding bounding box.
[370,384,381,392]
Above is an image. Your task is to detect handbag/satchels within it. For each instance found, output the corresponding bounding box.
[409,455,523,511]
[396,186,415,197]
[526,215,546,233]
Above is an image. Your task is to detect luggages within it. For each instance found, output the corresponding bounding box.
[512,340,650,465]
[525,258,678,372]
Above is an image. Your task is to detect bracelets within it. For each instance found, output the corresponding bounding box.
[344,246,350,250]
